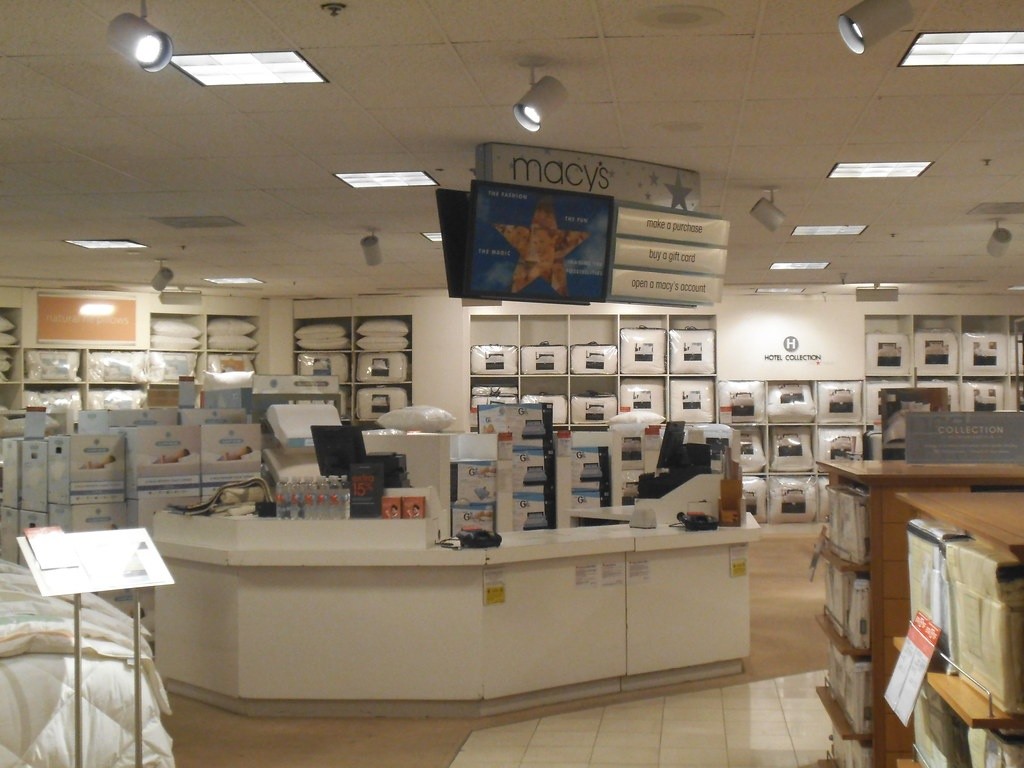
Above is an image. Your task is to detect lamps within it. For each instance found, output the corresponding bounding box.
[512,60,566,133]
[105,0,173,73]
[985,216,1012,259]
[360,228,384,266]
[837,0,913,55]
[149,258,175,291]
[749,185,787,233]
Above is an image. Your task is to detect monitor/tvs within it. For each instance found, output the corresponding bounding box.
[657,421,685,468]
[436,178,615,305]
[310,425,367,476]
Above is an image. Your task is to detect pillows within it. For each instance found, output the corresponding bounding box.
[150,321,201,338]
[150,335,201,349]
[0,349,14,382]
[296,337,351,350]
[0,315,16,333]
[356,336,409,350]
[0,332,17,345]
[207,317,257,336]
[207,334,258,351]
[377,403,455,432]
[356,318,409,337]
[295,323,346,340]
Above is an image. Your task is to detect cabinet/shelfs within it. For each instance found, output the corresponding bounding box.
[0,307,261,416]
[293,315,413,422]
[467,312,1024,531]
[811,460,1023,768]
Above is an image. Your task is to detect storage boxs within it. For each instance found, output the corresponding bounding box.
[450,400,612,540]
[1,371,343,572]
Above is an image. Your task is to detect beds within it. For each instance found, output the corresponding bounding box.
[0,557,177,768]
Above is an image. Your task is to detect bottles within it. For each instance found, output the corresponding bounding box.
[276,474,351,520]
